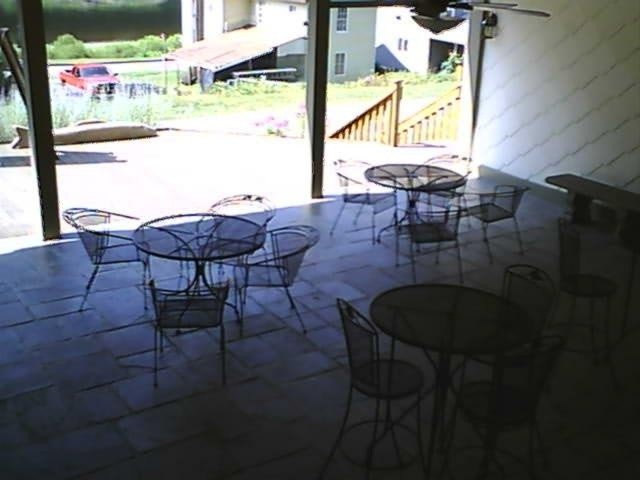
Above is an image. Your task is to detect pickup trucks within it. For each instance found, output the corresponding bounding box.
[58,61,125,101]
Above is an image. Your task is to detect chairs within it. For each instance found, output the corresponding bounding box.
[545,216,618,365]
[329,160,399,246]
[320,296,428,479]
[421,263,560,480]
[231,224,321,339]
[62,207,156,312]
[142,279,230,389]
[394,186,467,287]
[197,195,277,289]
[453,184,531,266]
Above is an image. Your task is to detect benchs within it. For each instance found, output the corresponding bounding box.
[544,174,639,248]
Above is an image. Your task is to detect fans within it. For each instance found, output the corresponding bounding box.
[322,0,551,33]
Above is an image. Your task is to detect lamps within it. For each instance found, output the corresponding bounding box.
[479,13,498,39]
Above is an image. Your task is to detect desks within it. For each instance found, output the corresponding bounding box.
[365,163,468,245]
[133,213,267,337]
[367,285,538,464]
